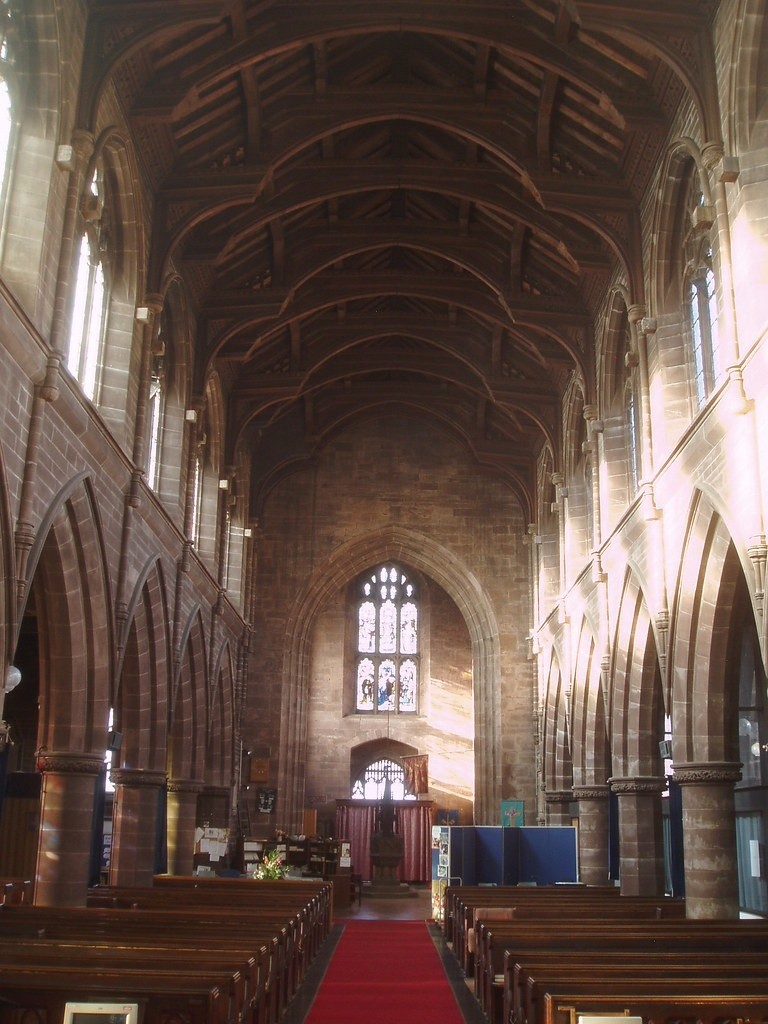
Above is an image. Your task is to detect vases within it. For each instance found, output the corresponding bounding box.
[277,836,282,841]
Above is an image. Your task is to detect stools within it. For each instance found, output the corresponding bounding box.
[350,880,361,905]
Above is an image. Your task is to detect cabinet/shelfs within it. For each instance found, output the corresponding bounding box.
[244,841,352,908]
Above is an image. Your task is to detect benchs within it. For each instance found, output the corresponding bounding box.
[0,875,336,1024]
[442,884,768,1024]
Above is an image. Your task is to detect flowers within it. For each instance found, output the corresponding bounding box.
[252,849,289,882]
[272,828,288,838]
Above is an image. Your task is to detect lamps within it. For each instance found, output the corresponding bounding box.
[240,784,250,792]
